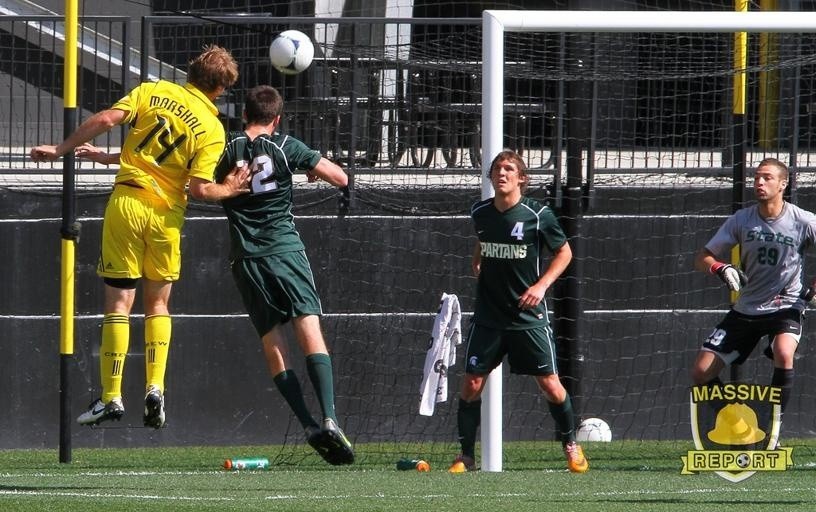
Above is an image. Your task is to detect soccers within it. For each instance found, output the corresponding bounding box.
[577,418,613,442]
[269,29,314,74]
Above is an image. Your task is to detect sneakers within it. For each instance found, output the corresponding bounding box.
[304,419,353,465]
[447,457,476,472]
[563,441,588,473]
[143,389,166,428]
[77,395,125,426]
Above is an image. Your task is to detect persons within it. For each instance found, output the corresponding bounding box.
[688,156,816,477]
[67,81,356,465]
[31,43,254,430]
[443,145,591,475]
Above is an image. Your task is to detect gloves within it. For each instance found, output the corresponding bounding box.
[801,281,816,307]
[715,264,748,290]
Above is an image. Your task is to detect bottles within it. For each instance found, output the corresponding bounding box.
[224,456,271,471]
[395,458,431,471]
